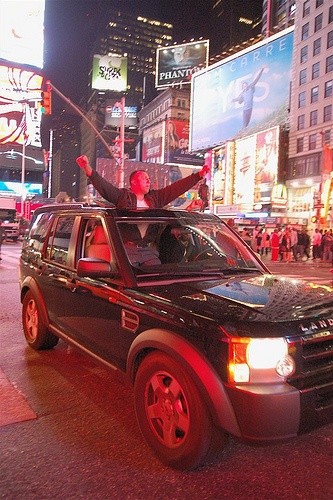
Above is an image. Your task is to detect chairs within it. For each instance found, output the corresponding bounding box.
[88,225,118,273]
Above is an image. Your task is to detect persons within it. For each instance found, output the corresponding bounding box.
[239,223,333,263]
[75,155,210,270]
[159,227,205,264]
[199,177,209,212]
[230,67,269,129]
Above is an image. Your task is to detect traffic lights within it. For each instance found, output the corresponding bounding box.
[41,91,52,115]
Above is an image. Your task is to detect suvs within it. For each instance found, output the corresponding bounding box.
[18,202,333,472]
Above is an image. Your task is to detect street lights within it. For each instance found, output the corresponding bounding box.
[21,141,44,215]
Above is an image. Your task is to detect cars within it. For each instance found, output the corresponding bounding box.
[19,216,30,235]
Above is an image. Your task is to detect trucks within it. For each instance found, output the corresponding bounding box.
[0,198,20,242]
